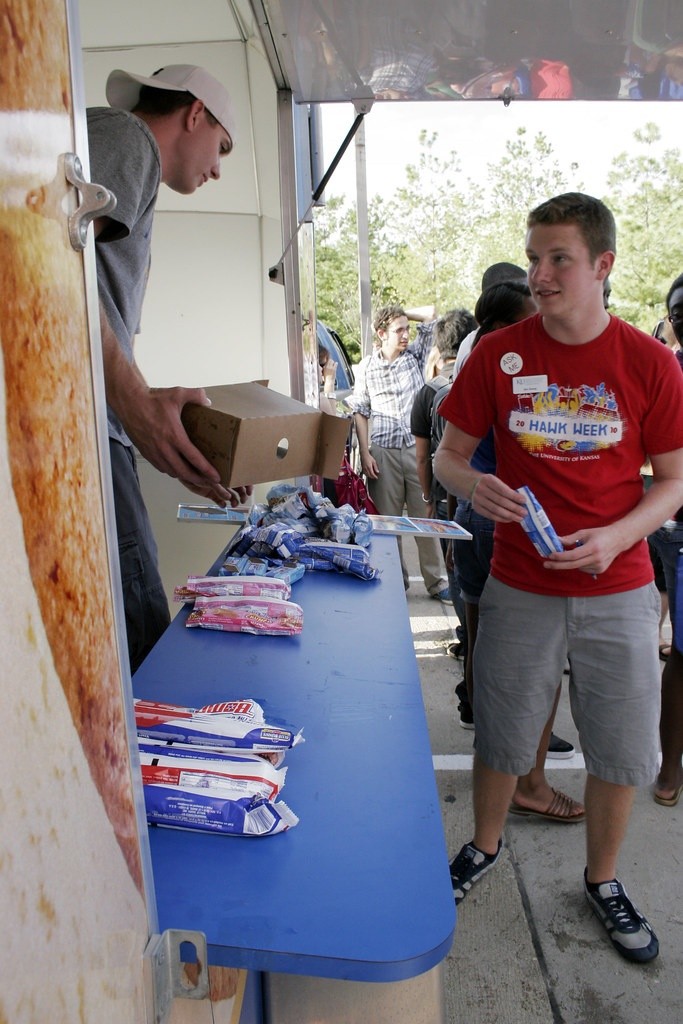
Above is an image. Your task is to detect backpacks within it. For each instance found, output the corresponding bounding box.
[424,374,453,503]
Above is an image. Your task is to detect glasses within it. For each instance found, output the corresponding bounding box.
[668,314,683,323]
[320,363,326,367]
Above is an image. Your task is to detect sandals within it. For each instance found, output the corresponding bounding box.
[660,644,671,661]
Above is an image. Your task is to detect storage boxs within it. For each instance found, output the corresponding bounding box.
[181,378,350,488]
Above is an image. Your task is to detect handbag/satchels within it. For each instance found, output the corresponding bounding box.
[334,457,380,515]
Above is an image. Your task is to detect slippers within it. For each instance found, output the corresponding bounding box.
[507,788,586,821]
[653,786,681,805]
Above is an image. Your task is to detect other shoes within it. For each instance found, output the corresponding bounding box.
[459,708,475,728]
[456,625,466,643]
[548,732,575,759]
[447,642,465,660]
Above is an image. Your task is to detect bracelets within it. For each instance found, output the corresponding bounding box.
[421,492,429,505]
[325,393,336,399]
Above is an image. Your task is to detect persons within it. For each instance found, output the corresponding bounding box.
[316,192,683,963]
[432,191,683,963]
[86,65,253,679]
[351,305,454,606]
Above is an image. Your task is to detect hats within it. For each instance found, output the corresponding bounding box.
[482,262,529,291]
[107,64,237,155]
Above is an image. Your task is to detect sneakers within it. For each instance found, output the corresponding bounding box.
[583,864,660,961]
[432,588,454,605]
[449,836,503,904]
